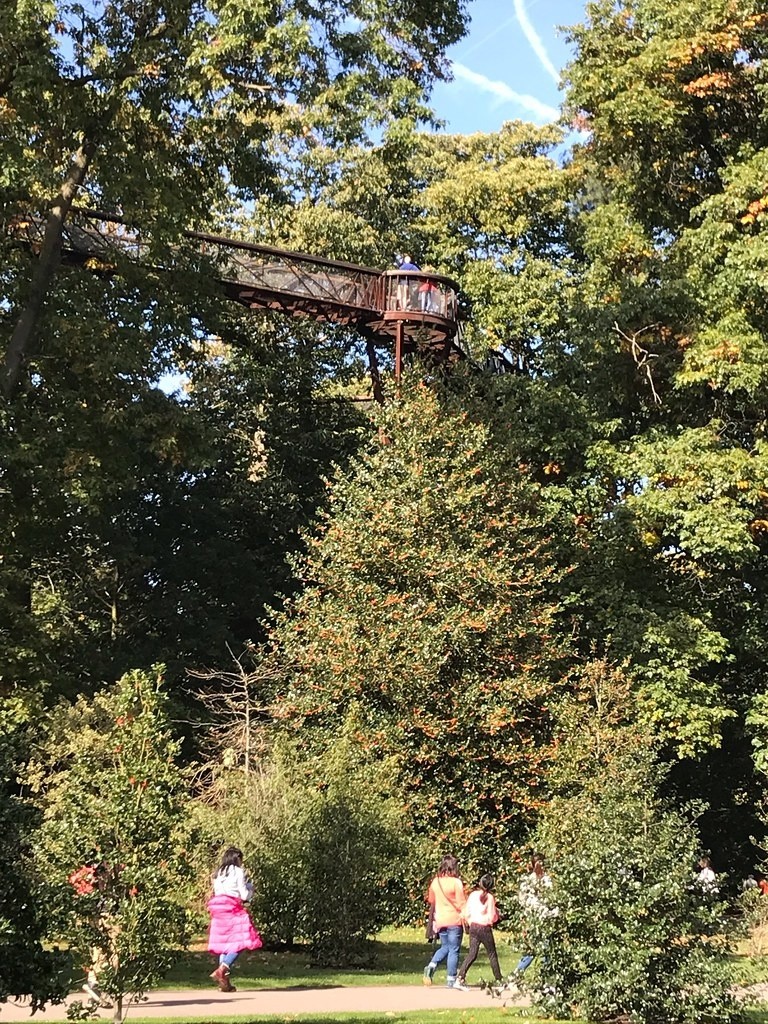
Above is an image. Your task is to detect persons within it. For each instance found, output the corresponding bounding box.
[422,856,469,988]
[70,844,137,1009]
[453,874,505,992]
[743,874,768,907]
[396,254,456,317]
[508,852,559,991]
[696,856,719,928]
[208,847,256,993]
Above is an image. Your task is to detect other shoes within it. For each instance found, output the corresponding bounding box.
[454,977,471,992]
[447,981,454,987]
[82,981,111,1008]
[423,966,435,986]
[508,971,524,982]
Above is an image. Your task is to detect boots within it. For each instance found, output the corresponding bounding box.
[209,963,237,992]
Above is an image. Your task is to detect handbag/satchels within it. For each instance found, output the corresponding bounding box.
[462,920,471,933]
